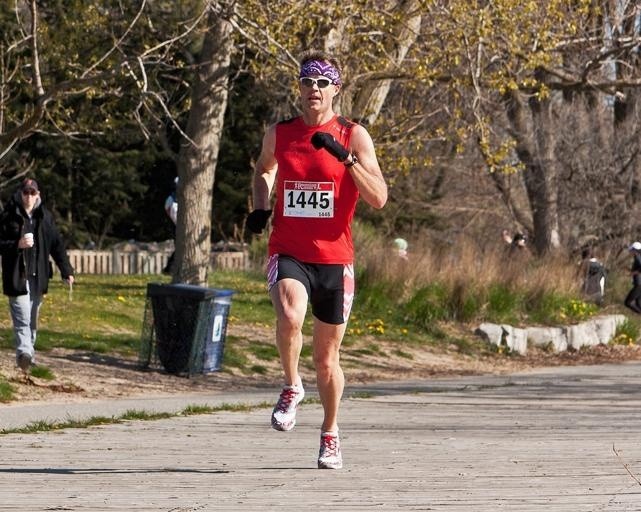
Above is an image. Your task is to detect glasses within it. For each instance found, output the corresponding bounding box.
[298,76,334,88]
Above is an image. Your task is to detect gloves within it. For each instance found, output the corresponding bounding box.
[310,131,349,161]
[246,206,273,235]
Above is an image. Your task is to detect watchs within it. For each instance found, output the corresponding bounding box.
[343,152,359,169]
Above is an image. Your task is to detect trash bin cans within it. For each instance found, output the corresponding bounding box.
[146,282,237,378]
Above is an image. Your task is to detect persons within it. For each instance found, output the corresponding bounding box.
[389,238,409,262]
[160,176,179,279]
[243,51,389,471]
[501,228,641,319]
[0,178,76,371]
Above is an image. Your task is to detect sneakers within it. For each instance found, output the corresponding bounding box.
[316,424,342,470]
[271,374,305,432]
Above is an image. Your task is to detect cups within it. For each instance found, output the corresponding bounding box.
[23,233,34,248]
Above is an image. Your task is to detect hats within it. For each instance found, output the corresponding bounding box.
[21,178,37,193]
